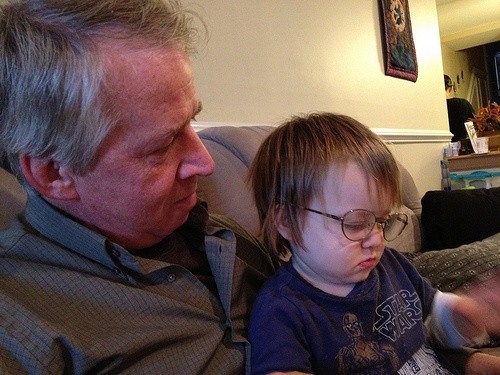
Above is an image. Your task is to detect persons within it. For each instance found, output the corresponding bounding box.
[249,114,490,375]
[0,0,500,375]
[444,75,475,142]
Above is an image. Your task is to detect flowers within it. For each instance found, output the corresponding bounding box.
[466,100,500,139]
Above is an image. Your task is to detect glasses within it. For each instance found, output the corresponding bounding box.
[294,205,409,242]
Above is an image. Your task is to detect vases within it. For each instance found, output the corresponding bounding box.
[477,130,500,151]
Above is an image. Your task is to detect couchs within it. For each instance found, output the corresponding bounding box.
[0,125,500,362]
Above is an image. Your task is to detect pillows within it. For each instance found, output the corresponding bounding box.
[410,186,500,348]
